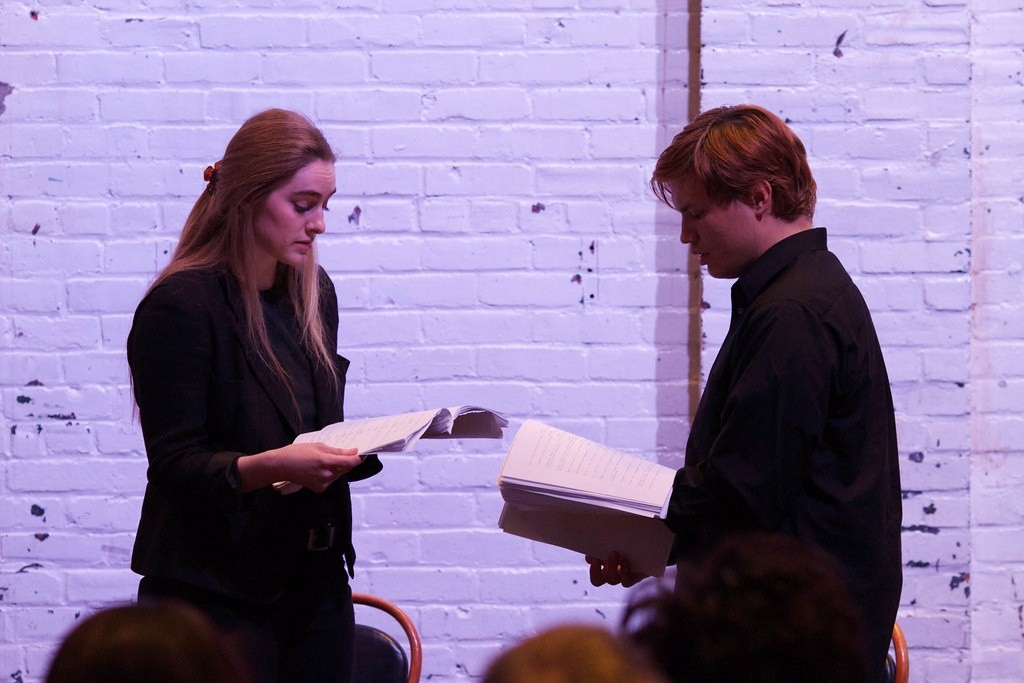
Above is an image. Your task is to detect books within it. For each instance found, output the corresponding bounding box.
[272,403,509,499]
[495,419,682,580]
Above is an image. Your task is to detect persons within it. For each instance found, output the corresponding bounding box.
[43,601,248,683]
[585,103,902,683]
[127,109,382,683]
[480,623,663,683]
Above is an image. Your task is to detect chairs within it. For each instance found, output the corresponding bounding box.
[352,591,422,683]
